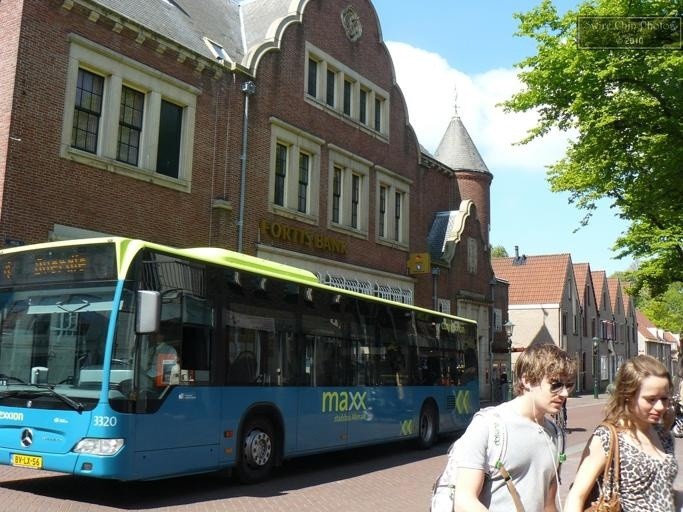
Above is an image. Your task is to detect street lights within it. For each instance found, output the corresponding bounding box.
[592,337,600,398]
[503,321,516,401]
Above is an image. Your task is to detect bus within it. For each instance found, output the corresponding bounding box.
[0,236,481,485]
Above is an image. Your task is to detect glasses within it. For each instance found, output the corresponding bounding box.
[549,380,577,393]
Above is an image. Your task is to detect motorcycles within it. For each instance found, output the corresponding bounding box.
[663,395,683,438]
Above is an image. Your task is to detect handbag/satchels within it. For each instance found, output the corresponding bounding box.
[569,422,622,512]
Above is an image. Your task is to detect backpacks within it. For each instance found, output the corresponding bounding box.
[429,405,507,512]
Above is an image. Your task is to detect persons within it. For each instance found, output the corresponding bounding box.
[450,342,578,512]
[120,328,177,402]
[562,354,680,512]
